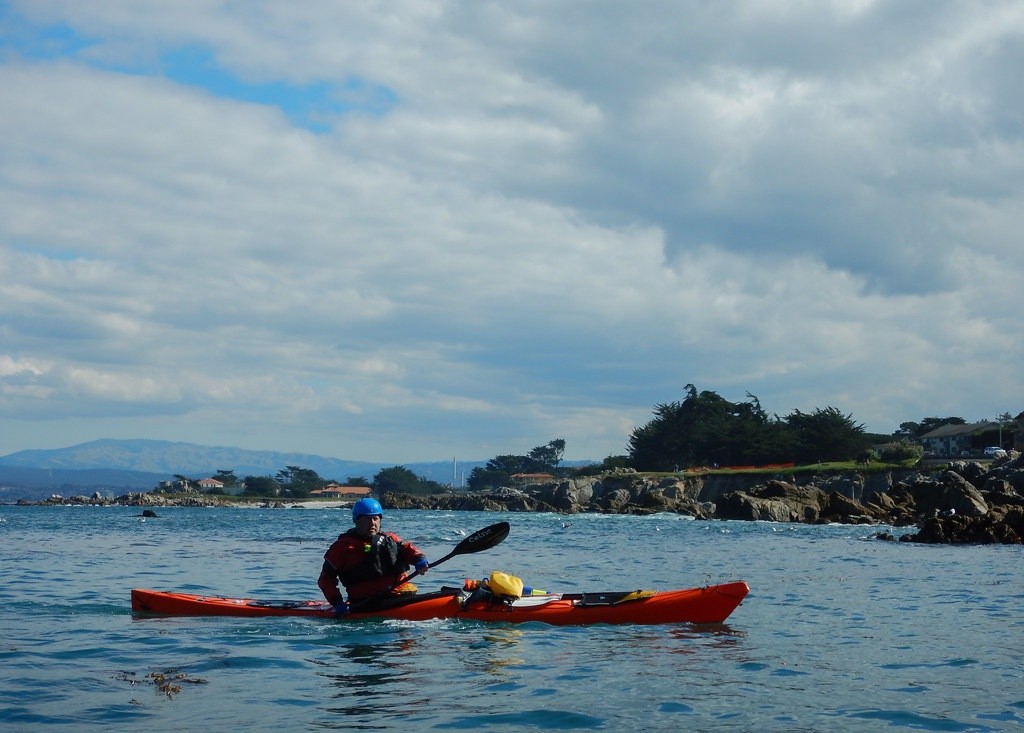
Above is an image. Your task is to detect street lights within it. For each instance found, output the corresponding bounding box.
[999,423,1005,449]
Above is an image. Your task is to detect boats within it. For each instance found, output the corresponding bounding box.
[128,572,751,625]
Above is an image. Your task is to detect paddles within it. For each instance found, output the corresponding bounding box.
[330,521,511,619]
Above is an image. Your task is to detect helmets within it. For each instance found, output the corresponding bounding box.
[351,498,383,523]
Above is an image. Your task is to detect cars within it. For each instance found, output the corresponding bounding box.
[984,447,1005,454]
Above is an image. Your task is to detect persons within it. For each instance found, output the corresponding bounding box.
[318,498,428,618]
